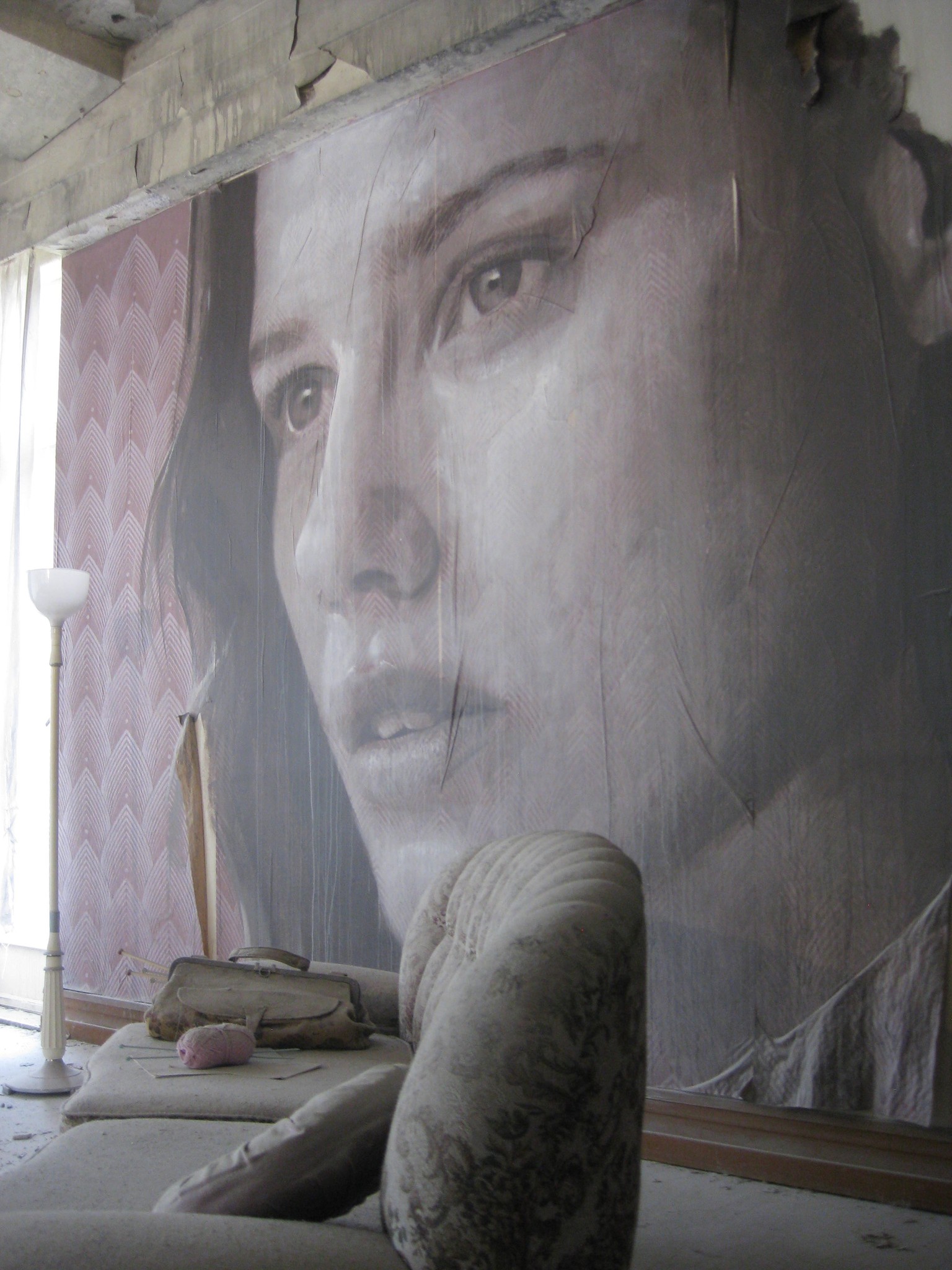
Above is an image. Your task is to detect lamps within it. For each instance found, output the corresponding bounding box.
[1,567,91,1097]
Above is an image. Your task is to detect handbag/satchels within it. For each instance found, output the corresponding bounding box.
[145,948,378,1052]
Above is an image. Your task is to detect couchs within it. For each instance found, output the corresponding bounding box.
[1,829,647,1270]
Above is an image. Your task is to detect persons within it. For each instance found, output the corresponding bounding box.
[154,1,951,1142]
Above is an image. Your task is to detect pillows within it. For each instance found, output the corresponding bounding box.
[153,1058,406,1226]
